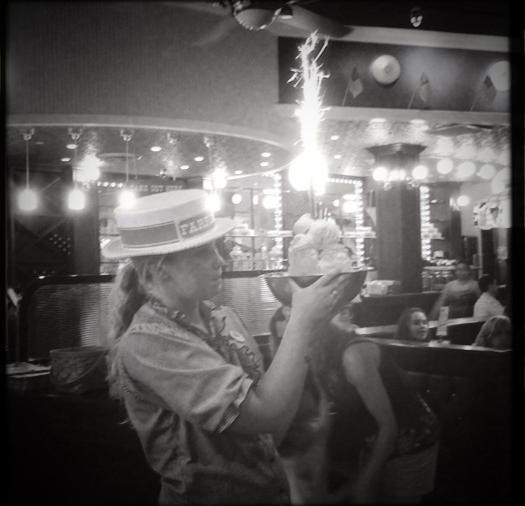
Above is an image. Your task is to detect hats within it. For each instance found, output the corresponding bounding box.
[100,191,234,259]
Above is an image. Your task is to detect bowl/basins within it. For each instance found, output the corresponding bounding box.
[265,263,374,314]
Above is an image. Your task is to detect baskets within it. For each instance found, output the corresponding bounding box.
[50,346,108,394]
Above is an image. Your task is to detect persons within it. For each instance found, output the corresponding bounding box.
[472,315,512,347]
[430,261,481,320]
[268,306,441,505]
[473,274,506,322]
[100,188,349,505]
[394,306,431,342]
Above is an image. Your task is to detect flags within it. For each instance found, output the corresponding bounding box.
[419,74,431,101]
[350,68,363,98]
[482,76,497,103]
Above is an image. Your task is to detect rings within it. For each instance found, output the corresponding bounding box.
[333,290,338,297]
